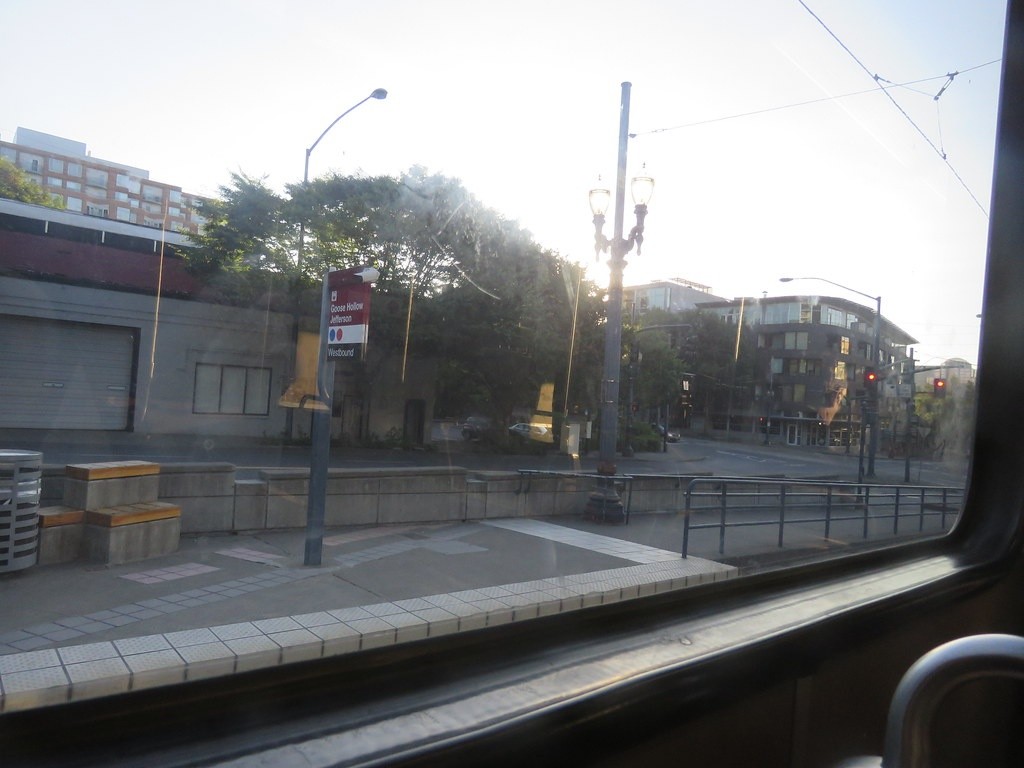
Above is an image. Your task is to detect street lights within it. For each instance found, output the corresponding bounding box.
[779,278,881,479]
[584,81,654,524]
[284,87,388,445]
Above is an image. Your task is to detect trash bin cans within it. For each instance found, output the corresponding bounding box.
[0,450,43,573]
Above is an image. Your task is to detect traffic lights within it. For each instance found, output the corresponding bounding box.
[933,378,946,398]
[759,417,764,422]
[864,366,878,389]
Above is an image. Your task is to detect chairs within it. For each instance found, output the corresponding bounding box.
[882,633,1024,768]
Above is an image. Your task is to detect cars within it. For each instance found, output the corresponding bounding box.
[509,423,548,440]
[460,417,526,444]
[650,423,680,443]
